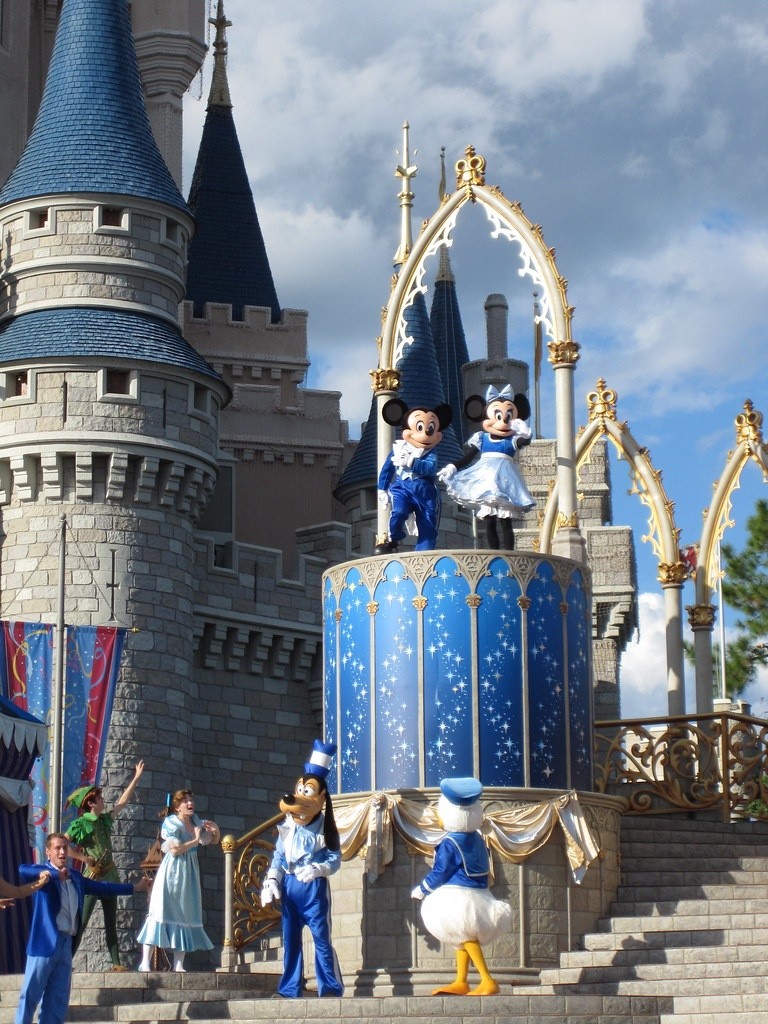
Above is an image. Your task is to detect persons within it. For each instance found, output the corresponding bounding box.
[15,834,151,1024]
[0,870,52,910]
[63,757,146,972]
[136,790,220,973]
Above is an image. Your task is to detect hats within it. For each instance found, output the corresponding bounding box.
[66,784,96,808]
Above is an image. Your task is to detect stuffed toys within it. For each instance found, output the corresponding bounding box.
[436,383,536,551]
[374,399,454,555]
[410,778,514,995]
[261,738,345,996]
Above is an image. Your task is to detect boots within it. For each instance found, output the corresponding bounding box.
[173,950,187,972]
[138,944,155,972]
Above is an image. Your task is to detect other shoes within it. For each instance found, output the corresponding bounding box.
[112,965,129,972]
